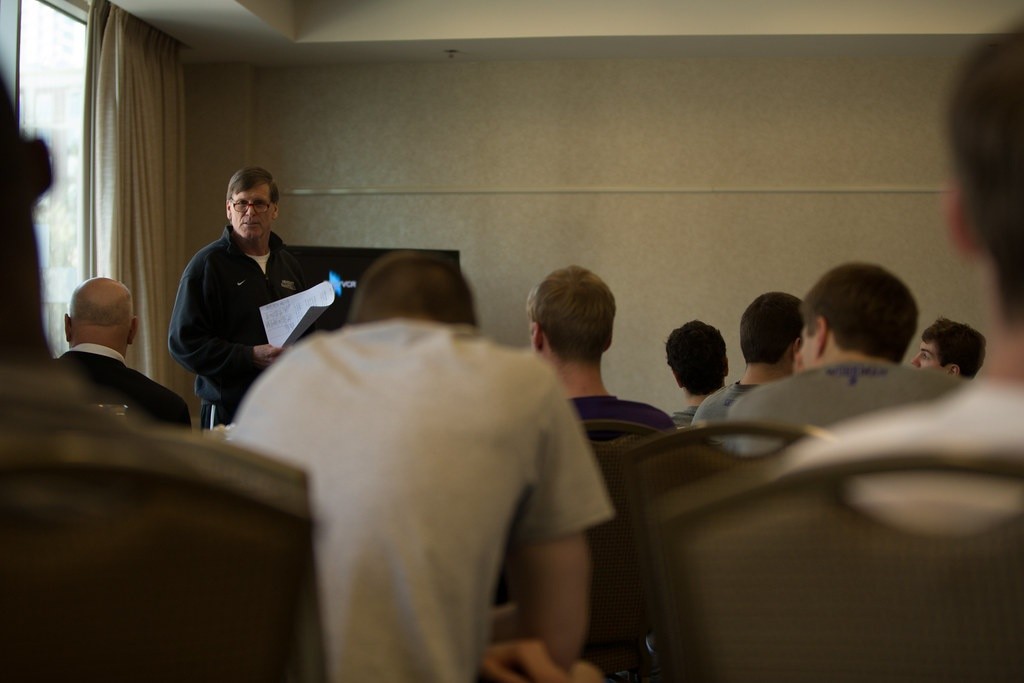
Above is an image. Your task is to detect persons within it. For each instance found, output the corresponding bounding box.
[666,12,1024,534]
[227,250,677,683]
[0,55,325,683]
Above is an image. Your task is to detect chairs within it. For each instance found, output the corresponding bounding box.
[1,365,1024,683]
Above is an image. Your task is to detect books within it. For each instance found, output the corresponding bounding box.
[259,281,335,349]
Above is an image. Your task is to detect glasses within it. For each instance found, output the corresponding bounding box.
[230,197,272,214]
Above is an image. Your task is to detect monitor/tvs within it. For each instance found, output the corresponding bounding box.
[288,244,459,329]
